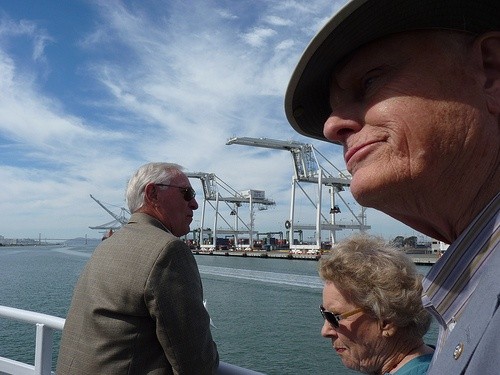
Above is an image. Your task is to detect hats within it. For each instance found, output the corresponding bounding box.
[285,0,500,146]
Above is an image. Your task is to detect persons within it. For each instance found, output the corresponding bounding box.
[311,233,436,375]
[284,2,500,375]
[50,163,222,375]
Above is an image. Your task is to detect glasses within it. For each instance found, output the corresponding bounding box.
[319,304,361,328]
[142,184,196,201]
